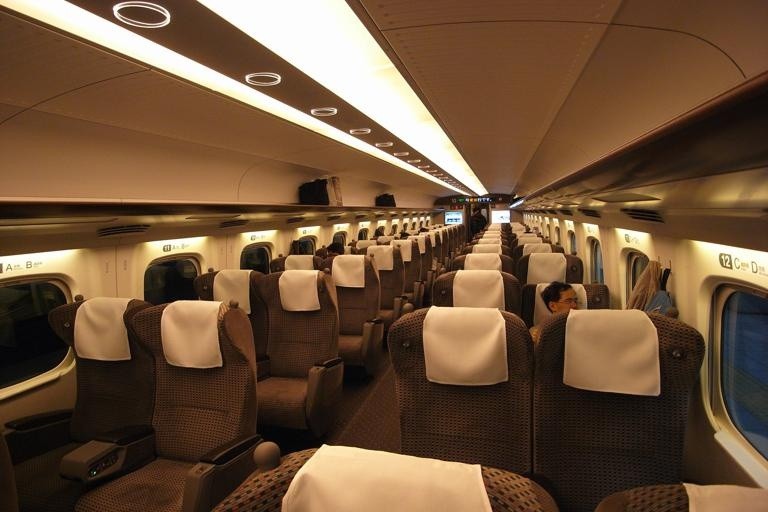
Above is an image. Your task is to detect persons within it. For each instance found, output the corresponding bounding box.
[470,207,487,237]
[527,280,579,345]
[326,242,345,256]
